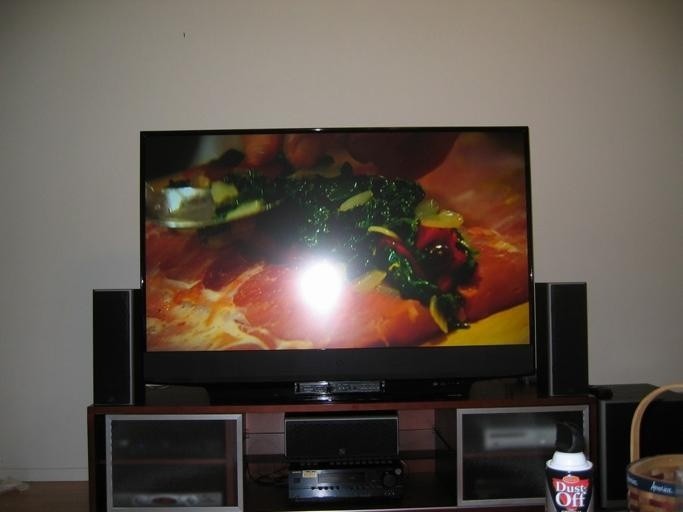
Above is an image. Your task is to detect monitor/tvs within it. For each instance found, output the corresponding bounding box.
[140,125,536,407]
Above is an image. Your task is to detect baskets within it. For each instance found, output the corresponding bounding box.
[626,384,683,512]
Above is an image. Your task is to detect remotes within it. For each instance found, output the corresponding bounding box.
[588,385,613,399]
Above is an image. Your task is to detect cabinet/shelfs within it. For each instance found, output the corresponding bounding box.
[86,383,600,512]
[592,384,683,512]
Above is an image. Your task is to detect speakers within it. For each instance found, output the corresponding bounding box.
[93,289,146,407]
[535,282,588,397]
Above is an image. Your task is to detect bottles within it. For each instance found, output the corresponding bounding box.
[543,447,597,512]
